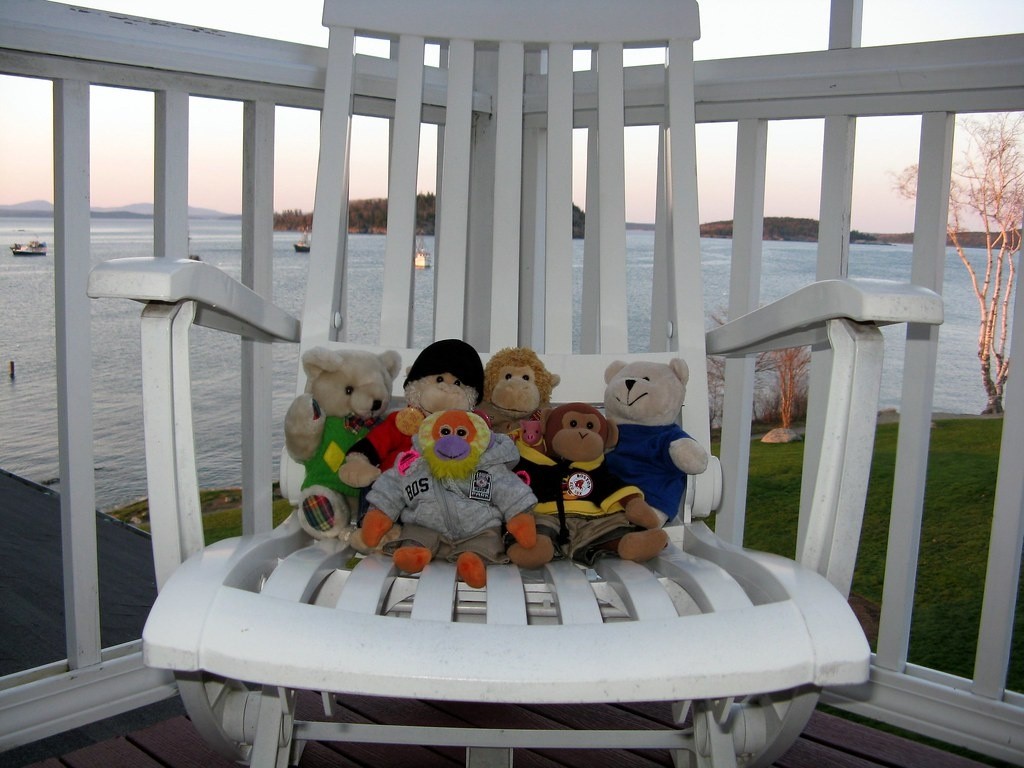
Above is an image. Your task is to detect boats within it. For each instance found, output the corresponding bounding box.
[9,240,48,256]
[293,226,310,253]
[414,237,433,267]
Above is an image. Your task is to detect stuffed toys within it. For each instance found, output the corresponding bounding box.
[507,402,669,569]
[338,339,484,553]
[604,358,708,528]
[362,411,537,589]
[520,419,546,454]
[285,347,402,539]
[478,347,560,442]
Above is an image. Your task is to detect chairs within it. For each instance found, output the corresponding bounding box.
[90,0,943,768]
